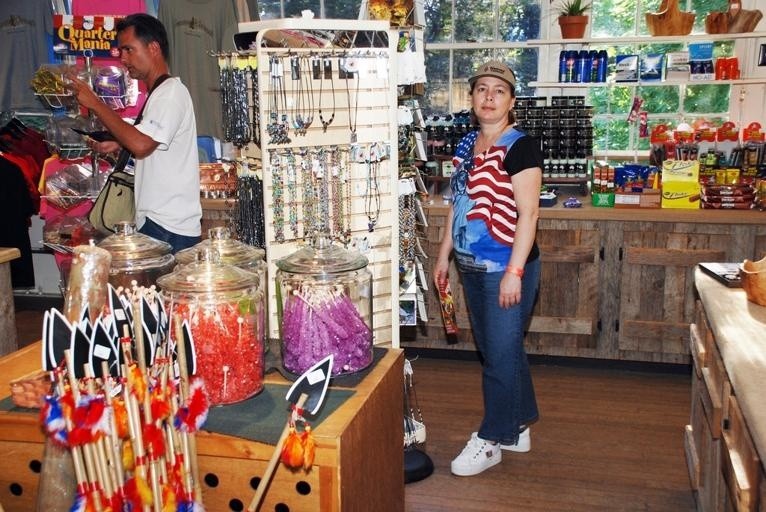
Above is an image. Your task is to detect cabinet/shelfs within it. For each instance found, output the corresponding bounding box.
[0,341,407,510]
[526,31,765,88]
[681,265,765,511]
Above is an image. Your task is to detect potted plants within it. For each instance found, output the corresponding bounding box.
[540,0,595,39]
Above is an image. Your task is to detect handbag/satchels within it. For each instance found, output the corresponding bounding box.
[86,171,134,237]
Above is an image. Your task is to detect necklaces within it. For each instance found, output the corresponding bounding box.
[217,53,421,273]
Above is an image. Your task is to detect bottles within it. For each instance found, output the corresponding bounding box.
[714,55,738,81]
[420,110,470,155]
[556,48,608,82]
[542,158,587,179]
[697,149,726,172]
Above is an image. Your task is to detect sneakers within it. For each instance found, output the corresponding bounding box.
[500,424,531,453]
[450,432,502,477]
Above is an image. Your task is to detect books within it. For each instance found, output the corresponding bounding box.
[696,261,747,289]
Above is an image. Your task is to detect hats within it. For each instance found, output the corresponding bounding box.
[468,61,516,89]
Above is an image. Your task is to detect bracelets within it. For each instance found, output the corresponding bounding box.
[505,263,525,278]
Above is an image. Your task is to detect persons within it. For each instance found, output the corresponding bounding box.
[68,13,202,257]
[434,62,544,476]
